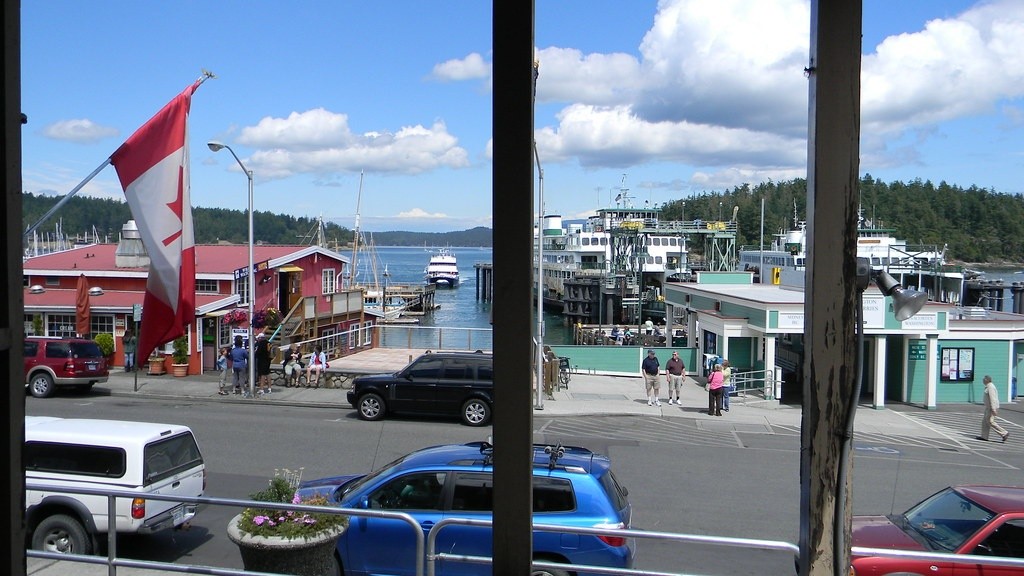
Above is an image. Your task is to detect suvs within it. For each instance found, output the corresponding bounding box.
[848,484,1024,576]
[23,415,208,556]
[347,349,493,428]
[294,440,638,576]
[23,334,109,397]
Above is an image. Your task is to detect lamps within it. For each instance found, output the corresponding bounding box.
[259,274,271,286]
[855,257,928,322]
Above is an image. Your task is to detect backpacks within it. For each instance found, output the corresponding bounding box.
[265,341,274,359]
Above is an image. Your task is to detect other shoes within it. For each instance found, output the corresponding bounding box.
[218,391,229,395]
[647,401,652,406]
[725,409,729,412]
[976,435,988,441]
[268,389,272,394]
[717,414,723,416]
[677,399,682,405]
[708,412,714,415]
[287,380,290,386]
[296,381,299,387]
[306,382,310,386]
[655,401,661,407]
[1003,433,1009,441]
[668,398,673,405]
[314,380,317,384]
[256,389,263,395]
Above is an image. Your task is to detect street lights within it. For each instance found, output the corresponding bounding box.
[207,141,258,394]
[533,139,547,410]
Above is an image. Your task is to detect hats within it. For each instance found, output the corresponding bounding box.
[255,333,264,338]
[648,349,656,354]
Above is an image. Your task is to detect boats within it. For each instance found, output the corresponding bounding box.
[358,220,406,322]
[736,188,947,285]
[533,187,693,321]
[425,249,460,287]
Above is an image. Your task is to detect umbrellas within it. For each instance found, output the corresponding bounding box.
[76,273,90,334]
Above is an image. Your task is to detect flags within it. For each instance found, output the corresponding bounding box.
[109,82,197,369]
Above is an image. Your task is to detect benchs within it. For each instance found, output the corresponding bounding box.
[270,364,354,389]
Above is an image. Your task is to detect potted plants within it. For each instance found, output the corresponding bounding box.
[171,335,189,377]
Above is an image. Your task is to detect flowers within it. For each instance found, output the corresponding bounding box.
[235,466,350,543]
[252,307,283,330]
[223,308,248,326]
[148,353,166,363]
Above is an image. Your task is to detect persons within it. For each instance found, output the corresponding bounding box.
[977,375,1009,441]
[306,346,326,384]
[644,317,653,335]
[425,350,432,354]
[231,340,249,394]
[707,364,723,416]
[673,256,678,267]
[721,360,730,412]
[642,349,662,406]
[122,329,136,372]
[256,340,272,394]
[216,347,228,395]
[654,322,661,337]
[283,343,304,388]
[578,318,632,345]
[474,350,483,353]
[665,351,686,405]
[232,333,273,395]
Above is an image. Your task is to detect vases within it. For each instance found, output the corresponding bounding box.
[147,358,166,375]
[227,513,349,576]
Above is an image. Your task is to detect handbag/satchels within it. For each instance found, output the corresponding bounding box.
[705,383,711,392]
